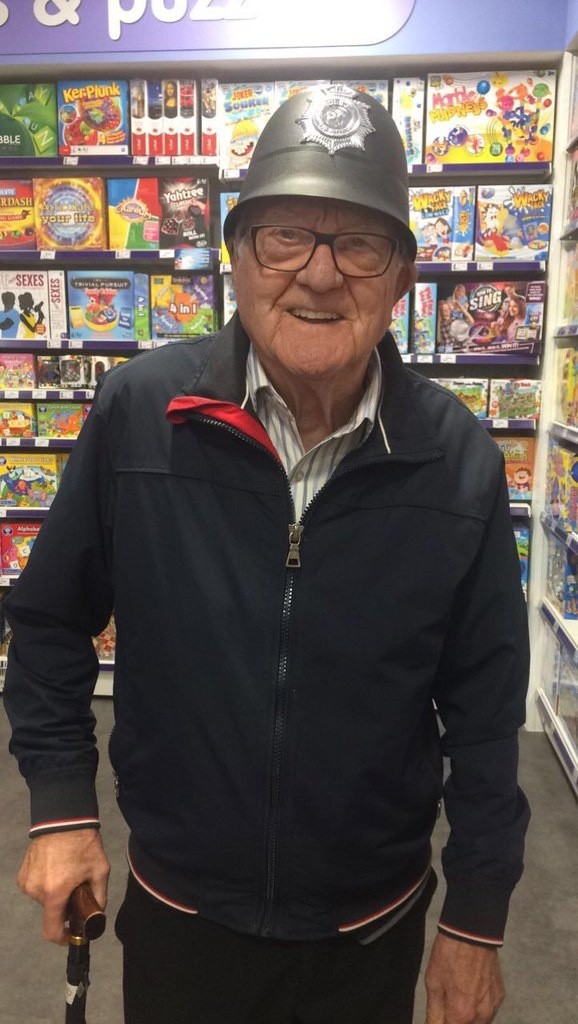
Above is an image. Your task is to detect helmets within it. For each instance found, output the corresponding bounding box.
[223,90,420,263]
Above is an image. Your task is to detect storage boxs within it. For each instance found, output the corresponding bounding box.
[0,69,557,665]
[540,52,578,758]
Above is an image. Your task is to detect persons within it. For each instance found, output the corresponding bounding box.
[435,283,526,342]
[0,84,530,1024]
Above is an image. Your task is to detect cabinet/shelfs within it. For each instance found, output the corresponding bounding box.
[523,34,578,794]
[0,30,563,698]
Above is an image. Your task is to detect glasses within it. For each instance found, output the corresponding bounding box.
[236,223,410,279]
